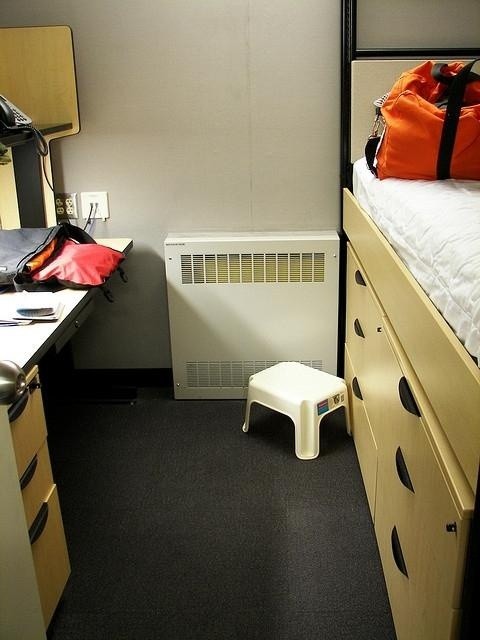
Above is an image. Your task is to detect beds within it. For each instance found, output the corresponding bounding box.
[338,57,479,638]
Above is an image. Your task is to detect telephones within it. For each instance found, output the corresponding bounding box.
[0,94,33,130]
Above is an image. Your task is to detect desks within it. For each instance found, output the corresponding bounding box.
[0,237,139,633]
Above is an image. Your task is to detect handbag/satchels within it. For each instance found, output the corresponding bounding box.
[0,224,129,303]
[365,60,480,180]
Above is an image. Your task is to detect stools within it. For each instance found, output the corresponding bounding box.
[239,361,351,463]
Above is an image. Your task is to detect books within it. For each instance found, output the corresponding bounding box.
[0,290,65,327]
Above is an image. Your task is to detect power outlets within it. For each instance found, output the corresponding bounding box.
[54,191,109,221]
